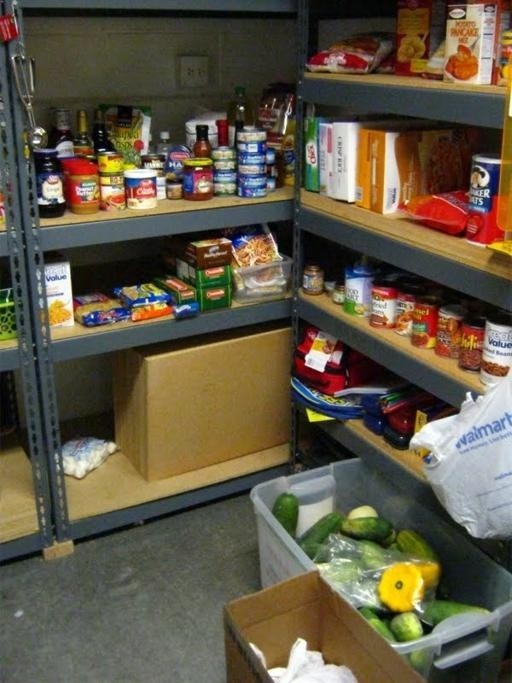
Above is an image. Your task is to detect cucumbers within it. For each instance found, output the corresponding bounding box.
[272,493,490,671]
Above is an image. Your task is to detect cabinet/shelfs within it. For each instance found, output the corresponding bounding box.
[294,1,512,522]
[0,0,295,564]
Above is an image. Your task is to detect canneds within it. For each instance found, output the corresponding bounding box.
[140,154,165,175]
[438,304,463,356]
[460,316,485,373]
[303,266,323,295]
[123,168,157,208]
[30,149,64,216]
[212,149,237,195]
[397,284,416,335]
[366,278,395,327]
[345,268,372,318]
[332,282,347,303]
[166,178,181,199]
[412,294,439,350]
[482,318,511,388]
[68,164,102,214]
[98,150,126,209]
[183,157,216,200]
[466,154,504,247]
[236,127,269,198]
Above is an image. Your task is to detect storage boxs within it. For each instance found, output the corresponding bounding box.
[223,570,424,682]
[105,328,295,483]
[250,458,511,679]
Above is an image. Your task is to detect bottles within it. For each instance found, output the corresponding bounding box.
[53,87,253,162]
